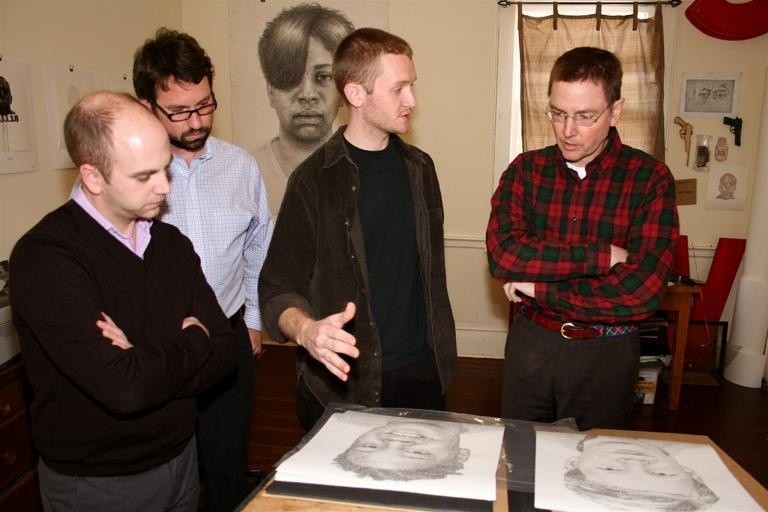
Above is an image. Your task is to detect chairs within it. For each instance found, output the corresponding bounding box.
[663,238,747,374]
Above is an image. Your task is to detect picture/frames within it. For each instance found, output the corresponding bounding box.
[44,65,102,171]
[0,60,39,176]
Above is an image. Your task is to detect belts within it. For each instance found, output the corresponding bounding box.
[516,301,636,339]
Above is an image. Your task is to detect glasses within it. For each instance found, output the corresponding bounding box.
[542,97,616,124]
[152,92,219,123]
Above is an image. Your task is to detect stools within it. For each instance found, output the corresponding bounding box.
[633,366,663,405]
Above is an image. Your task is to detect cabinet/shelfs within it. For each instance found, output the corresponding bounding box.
[1,354,43,512]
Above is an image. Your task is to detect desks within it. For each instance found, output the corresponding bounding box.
[235,402,768,512]
[508,275,707,411]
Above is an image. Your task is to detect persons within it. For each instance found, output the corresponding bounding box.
[691,80,734,112]
[486,46,680,432]
[247,2,356,226]
[258,27,458,436]
[70,27,274,511]
[563,433,720,512]
[716,173,737,200]
[331,416,471,481]
[7,89,240,512]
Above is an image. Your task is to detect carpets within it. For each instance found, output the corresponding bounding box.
[660,369,719,386]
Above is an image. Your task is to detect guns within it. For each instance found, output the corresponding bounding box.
[675,116,692,166]
[723,116,742,146]
